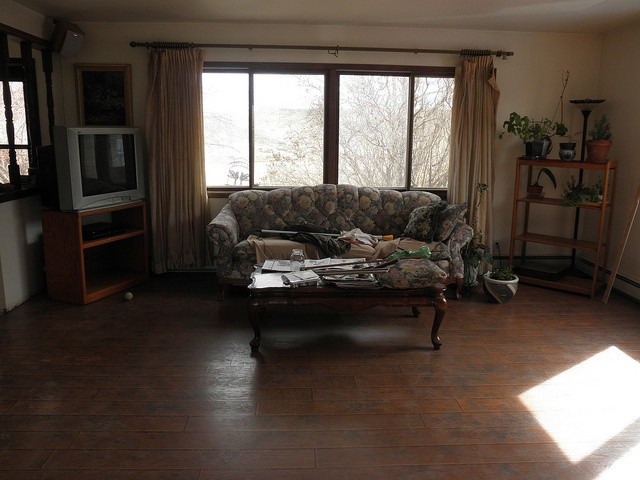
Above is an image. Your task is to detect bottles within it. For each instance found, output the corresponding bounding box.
[290,249,305,272]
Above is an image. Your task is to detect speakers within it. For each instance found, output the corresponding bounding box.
[51,22,85,59]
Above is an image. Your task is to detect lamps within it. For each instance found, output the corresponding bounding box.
[565,99,607,277]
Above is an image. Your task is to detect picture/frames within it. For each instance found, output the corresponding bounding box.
[74,64,133,128]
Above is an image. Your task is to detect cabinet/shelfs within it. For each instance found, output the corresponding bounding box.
[507,155,619,298]
[42,196,148,302]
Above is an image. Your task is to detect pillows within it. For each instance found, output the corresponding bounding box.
[401,203,447,242]
[439,202,468,240]
[373,257,449,289]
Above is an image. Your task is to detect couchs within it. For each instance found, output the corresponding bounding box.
[205,183,475,301]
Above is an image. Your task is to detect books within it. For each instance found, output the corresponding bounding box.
[261,257,395,290]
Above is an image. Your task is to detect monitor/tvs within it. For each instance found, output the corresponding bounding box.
[36,127,145,213]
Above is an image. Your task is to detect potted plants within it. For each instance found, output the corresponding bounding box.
[498,113,556,159]
[525,167,557,200]
[483,265,519,304]
[585,112,615,163]
[560,175,594,207]
[460,183,495,288]
[540,121,567,159]
[594,175,610,204]
[558,130,582,161]
